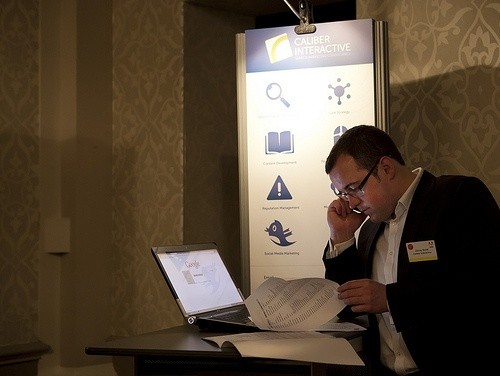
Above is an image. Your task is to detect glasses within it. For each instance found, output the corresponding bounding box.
[332,153,391,202]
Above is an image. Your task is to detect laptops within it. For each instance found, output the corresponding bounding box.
[150,242,271,332]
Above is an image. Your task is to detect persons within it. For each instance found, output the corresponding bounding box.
[322,125,500,376]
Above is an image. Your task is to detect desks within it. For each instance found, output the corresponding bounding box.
[84,325,366,376]
